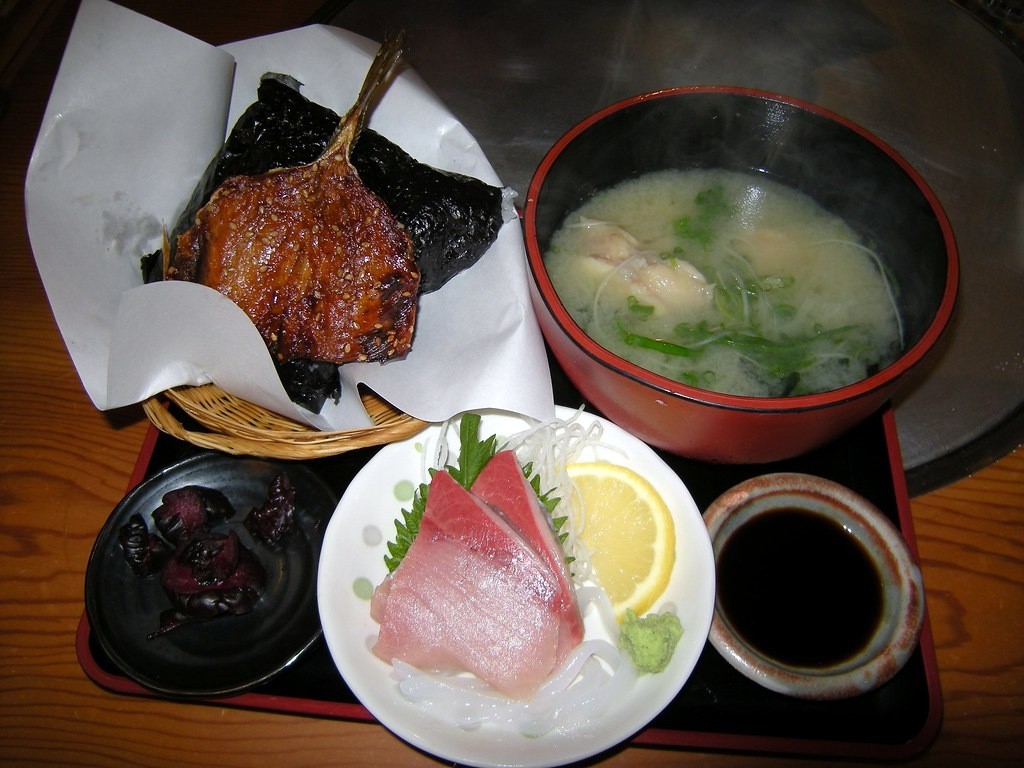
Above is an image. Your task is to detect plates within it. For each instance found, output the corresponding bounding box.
[701,472,925,699]
[84,451,338,696]
[316,404,716,768]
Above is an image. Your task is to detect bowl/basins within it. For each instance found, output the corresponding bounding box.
[522,84,960,466]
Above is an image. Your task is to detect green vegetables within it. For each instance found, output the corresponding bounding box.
[382,413,575,585]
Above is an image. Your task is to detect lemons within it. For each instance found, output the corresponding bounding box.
[554,463,676,624]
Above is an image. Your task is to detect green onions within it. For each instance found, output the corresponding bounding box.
[610,183,871,390]
